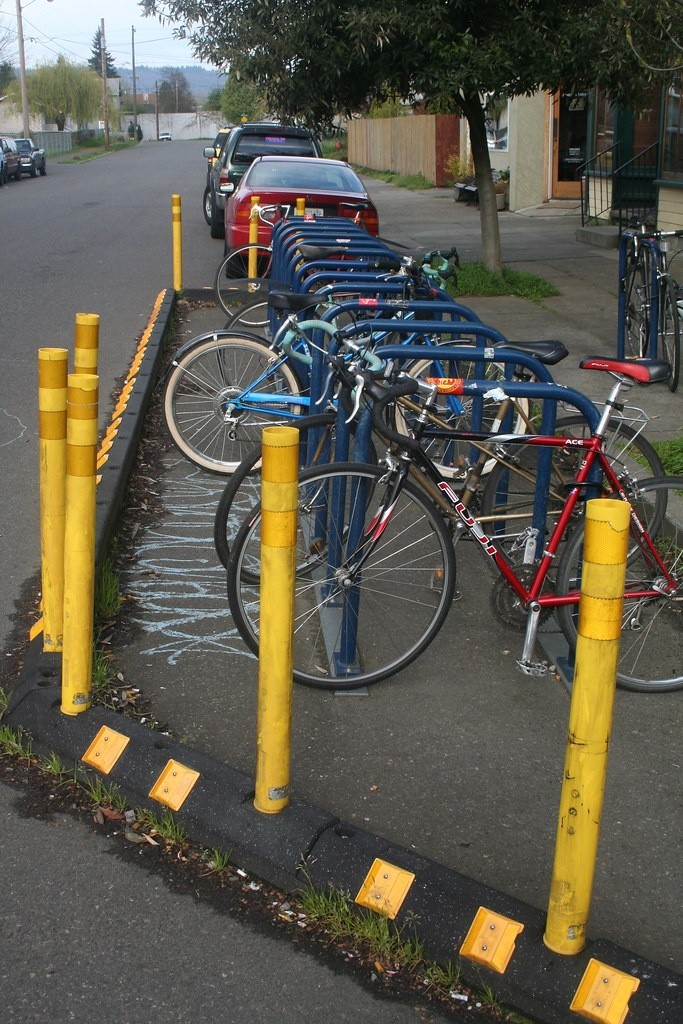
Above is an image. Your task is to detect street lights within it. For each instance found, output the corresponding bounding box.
[15,0,54,139]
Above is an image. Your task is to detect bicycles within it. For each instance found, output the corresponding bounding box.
[622,217,683,393]
[153,203,683,694]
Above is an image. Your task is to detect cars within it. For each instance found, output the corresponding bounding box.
[207,127,234,171]
[218,154,381,280]
[159,132,171,141]
[0,136,47,185]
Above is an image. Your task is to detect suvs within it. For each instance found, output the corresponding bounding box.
[202,122,325,239]
